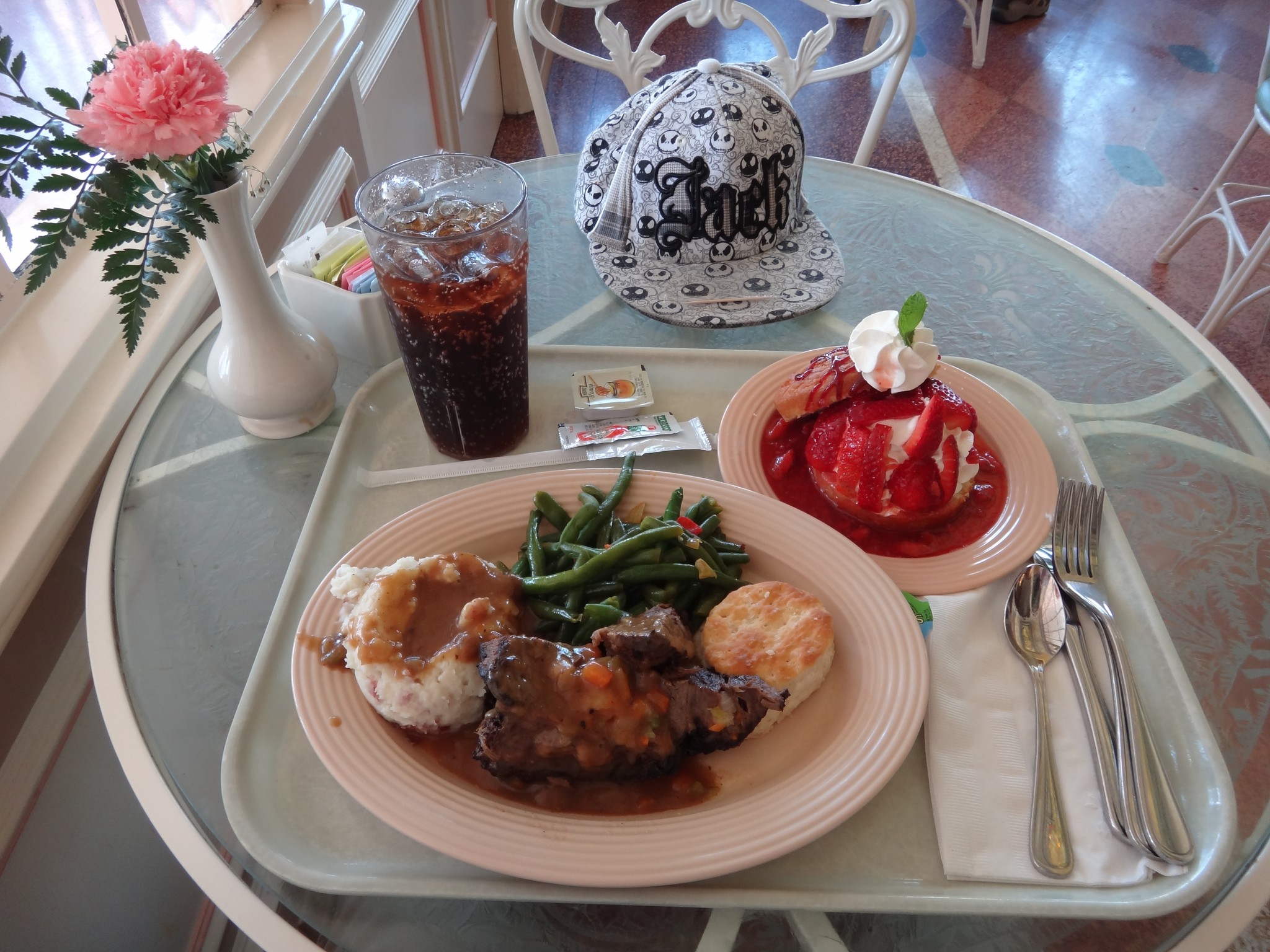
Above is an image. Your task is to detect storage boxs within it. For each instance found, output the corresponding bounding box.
[277,221,403,368]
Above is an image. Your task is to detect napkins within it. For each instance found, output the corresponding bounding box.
[923,563,1188,887]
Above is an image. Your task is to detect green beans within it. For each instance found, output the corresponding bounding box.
[495,451,753,651]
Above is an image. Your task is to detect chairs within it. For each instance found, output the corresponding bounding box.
[1155,34,1270,337]
[512,0,919,167]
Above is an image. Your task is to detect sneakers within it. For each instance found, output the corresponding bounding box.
[988,0,1051,25]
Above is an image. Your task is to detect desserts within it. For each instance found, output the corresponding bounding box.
[771,288,980,534]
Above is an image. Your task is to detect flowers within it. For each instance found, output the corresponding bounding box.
[0,31,270,359]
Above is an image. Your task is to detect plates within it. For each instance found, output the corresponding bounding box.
[291,467,930,887]
[718,346,1060,595]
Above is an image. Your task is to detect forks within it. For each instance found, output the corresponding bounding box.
[1052,477,1196,863]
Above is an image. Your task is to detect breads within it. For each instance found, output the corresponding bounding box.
[703,577,833,737]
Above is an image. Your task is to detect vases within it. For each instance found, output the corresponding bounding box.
[165,167,337,438]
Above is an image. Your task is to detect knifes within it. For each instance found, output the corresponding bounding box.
[1034,543,1132,848]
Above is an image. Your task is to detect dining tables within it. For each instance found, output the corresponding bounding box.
[85,152,1270,952]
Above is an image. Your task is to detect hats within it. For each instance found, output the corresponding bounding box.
[574,58,844,329]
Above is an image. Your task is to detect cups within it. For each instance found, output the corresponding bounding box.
[354,152,530,461]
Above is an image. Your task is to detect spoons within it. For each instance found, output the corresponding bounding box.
[1002,562,1077,879]
